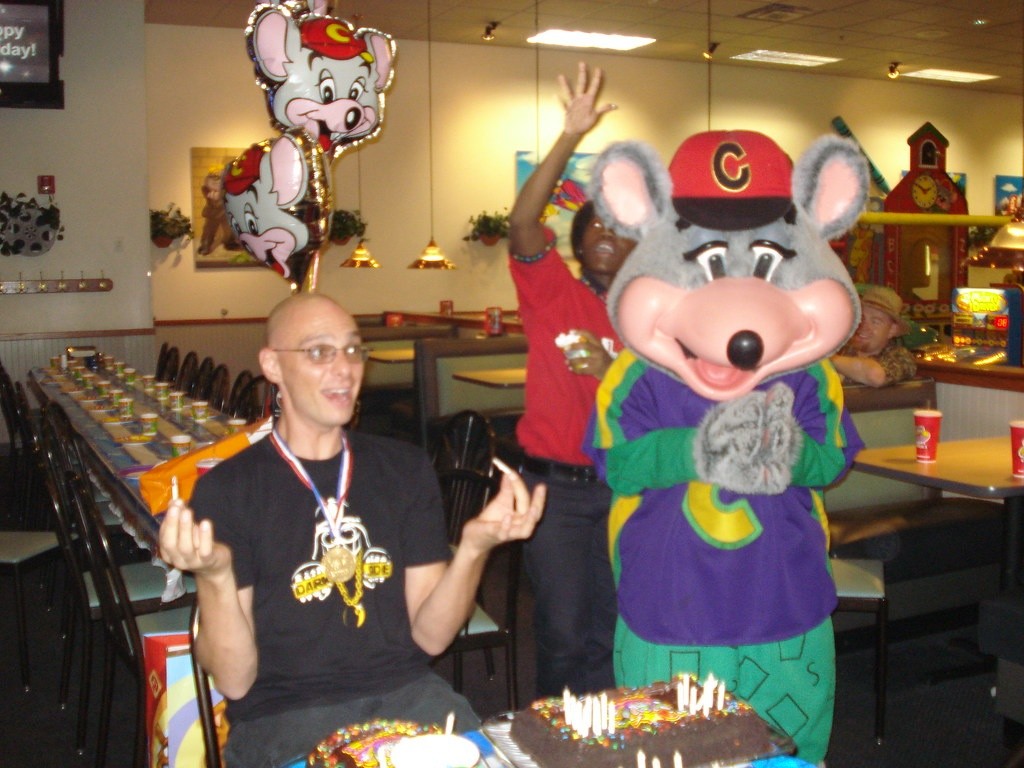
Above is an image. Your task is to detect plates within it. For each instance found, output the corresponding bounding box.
[98,416,139,425]
[115,436,153,446]
[186,408,219,419]
[39,350,191,412]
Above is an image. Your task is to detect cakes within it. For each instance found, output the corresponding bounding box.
[508,672,775,768]
[306,719,461,768]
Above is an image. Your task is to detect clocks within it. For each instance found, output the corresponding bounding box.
[912,175,938,208]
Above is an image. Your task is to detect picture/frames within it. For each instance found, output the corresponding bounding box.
[0,0,67,110]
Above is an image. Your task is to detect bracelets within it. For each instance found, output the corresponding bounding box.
[511,235,557,263]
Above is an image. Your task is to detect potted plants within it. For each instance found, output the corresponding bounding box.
[150,203,195,249]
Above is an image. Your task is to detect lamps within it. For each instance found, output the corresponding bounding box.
[887,63,900,82]
[701,41,719,61]
[340,143,381,270]
[406,0,458,276]
[477,19,501,40]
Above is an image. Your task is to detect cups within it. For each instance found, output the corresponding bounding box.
[169,435,191,457]
[140,413,158,435]
[118,398,133,418]
[227,420,247,436]
[1009,420,1024,478]
[914,411,943,463]
[192,402,209,422]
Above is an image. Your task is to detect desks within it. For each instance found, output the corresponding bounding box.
[367,348,414,364]
[849,435,1024,500]
[451,367,529,388]
[25,365,253,572]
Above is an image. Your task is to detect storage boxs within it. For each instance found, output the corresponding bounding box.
[145,636,231,768]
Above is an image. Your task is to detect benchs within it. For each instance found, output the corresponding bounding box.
[420,337,533,450]
[358,324,458,410]
[825,378,1007,586]
[392,340,419,438]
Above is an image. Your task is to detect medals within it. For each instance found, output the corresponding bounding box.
[321,546,357,583]
[341,604,365,631]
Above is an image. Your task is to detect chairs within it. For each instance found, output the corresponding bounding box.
[829,558,888,747]
[0,342,278,768]
[433,468,519,712]
[189,590,221,768]
[434,411,497,549]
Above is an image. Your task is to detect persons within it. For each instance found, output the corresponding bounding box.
[828,283,917,390]
[157,292,548,768]
[506,60,652,701]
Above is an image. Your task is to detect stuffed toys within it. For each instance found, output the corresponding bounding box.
[588,129,870,768]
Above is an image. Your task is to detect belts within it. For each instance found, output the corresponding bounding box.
[520,452,597,483]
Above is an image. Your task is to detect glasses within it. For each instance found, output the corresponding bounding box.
[273,345,373,365]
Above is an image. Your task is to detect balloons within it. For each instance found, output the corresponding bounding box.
[221,3,399,291]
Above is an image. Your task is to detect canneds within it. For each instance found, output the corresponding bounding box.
[484,306,503,338]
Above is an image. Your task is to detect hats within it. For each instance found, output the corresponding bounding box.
[856,282,910,337]
